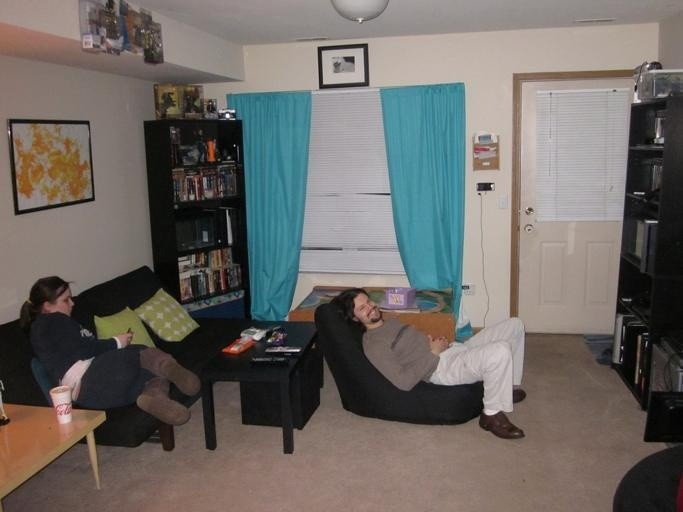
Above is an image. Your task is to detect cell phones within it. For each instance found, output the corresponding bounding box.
[127,327,131,332]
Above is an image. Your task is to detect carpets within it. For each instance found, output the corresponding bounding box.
[582,330,615,367]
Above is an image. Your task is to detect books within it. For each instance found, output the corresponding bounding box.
[629,218,655,276]
[175,246,243,303]
[378,300,422,313]
[610,313,649,397]
[172,169,238,202]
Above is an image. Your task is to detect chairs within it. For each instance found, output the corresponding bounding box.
[313,288,483,427]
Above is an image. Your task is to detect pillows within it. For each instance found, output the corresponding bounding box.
[133,286,201,343]
[93,305,157,352]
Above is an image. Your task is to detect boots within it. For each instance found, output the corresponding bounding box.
[135,377,190,427]
[142,347,200,396]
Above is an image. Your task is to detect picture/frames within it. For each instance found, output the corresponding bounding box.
[317,43,369,89]
[6,117,96,215]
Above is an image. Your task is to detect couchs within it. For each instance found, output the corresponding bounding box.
[0,266,253,452]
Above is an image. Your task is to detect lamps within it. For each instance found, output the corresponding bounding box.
[331,0,390,25]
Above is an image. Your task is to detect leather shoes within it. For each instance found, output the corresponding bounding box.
[479,410,525,439]
[511,388,526,403]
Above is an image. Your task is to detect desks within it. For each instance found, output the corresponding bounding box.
[194,320,325,453]
[0,402,108,509]
[288,284,456,341]
[611,446,682,511]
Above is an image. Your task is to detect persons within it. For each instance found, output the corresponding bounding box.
[17,276,200,425]
[335,286,528,440]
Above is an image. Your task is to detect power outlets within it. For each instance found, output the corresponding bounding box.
[463,284,474,297]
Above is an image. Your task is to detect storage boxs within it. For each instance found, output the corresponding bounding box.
[634,69,683,101]
[239,353,321,430]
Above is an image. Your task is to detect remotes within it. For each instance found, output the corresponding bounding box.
[250,357,288,365]
[264,346,301,355]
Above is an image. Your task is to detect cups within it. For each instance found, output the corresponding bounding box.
[49,386,71,424]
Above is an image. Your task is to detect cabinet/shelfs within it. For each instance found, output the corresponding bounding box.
[610,97,683,443]
[144,117,252,322]
